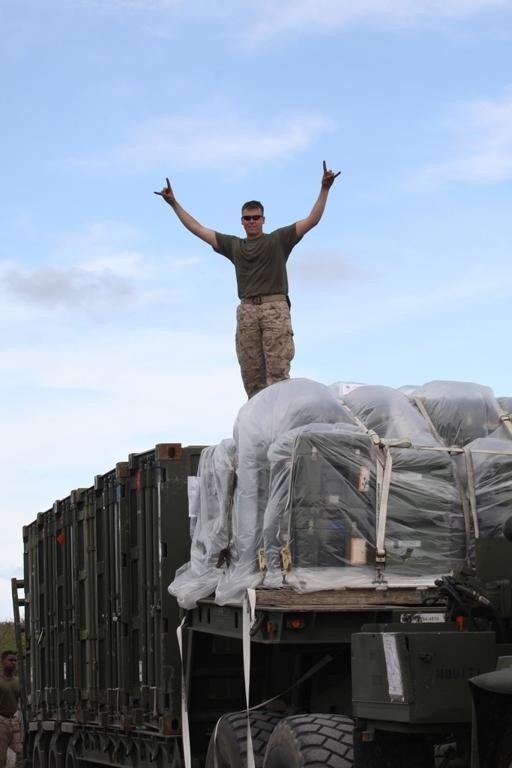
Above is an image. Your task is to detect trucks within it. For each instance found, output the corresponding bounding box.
[10,442,512,768]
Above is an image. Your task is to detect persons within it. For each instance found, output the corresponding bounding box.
[154,161,341,400]
[0,650,26,768]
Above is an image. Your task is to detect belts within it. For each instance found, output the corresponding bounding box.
[240,294,287,305]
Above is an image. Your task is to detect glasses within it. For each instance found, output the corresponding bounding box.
[241,215,263,221]
